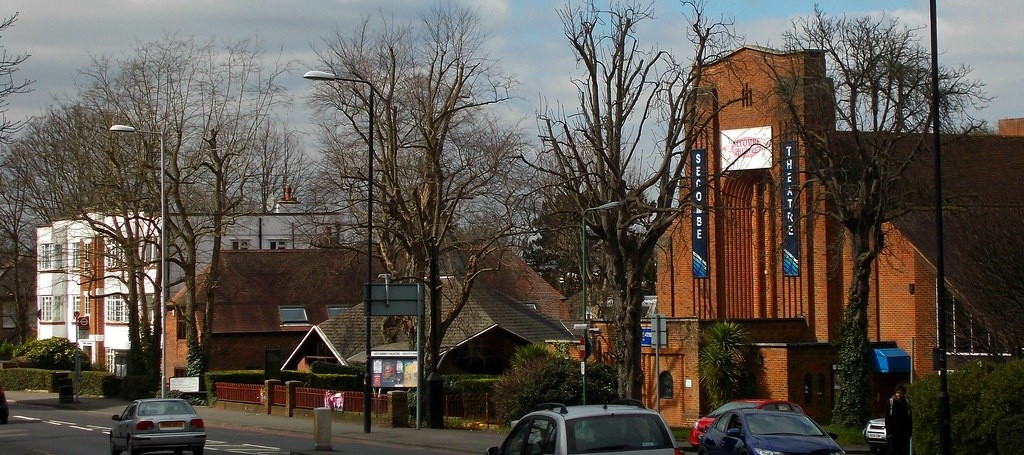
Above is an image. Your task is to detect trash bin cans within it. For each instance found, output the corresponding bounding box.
[314,406,332,451]
[58,378,75,403]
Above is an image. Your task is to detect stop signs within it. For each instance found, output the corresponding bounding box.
[79,317,89,329]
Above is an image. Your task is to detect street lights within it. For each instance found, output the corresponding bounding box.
[109,124,166,401]
[303,69,374,433]
[581,200,625,404]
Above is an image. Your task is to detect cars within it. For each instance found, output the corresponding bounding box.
[484,399,686,454]
[695,406,846,455]
[689,397,809,450]
[862,417,890,454]
[0,386,9,425]
[109,399,206,455]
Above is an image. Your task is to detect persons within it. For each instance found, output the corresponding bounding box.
[886,385,912,455]
